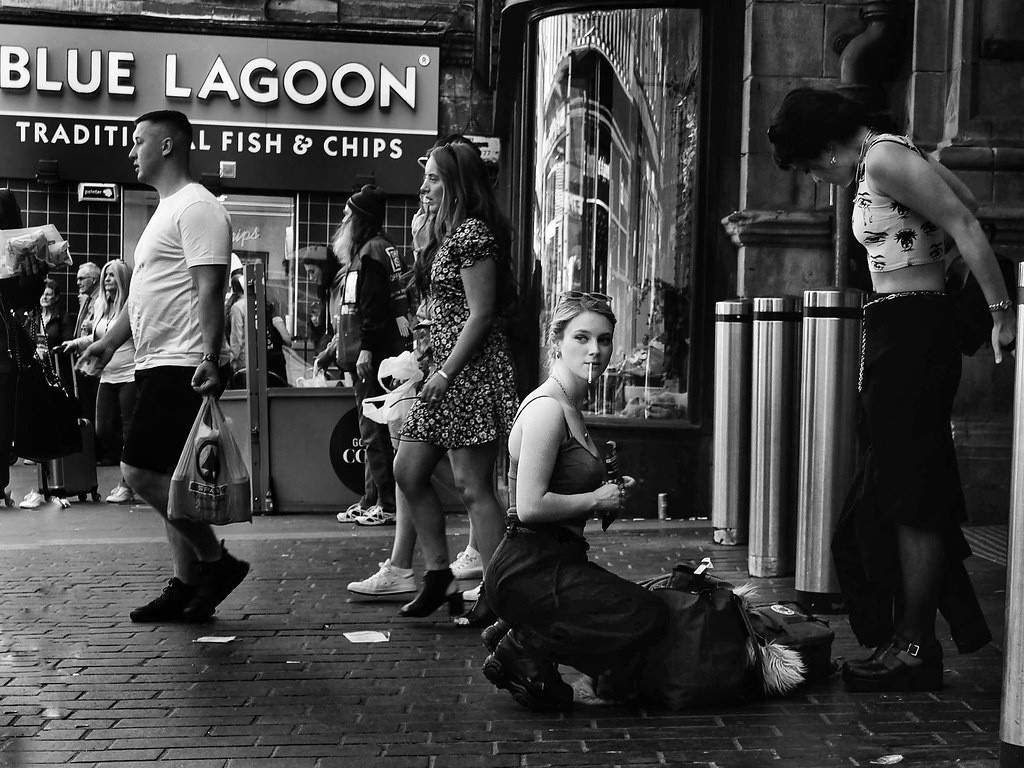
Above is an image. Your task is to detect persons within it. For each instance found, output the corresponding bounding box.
[391,142,521,626]
[62,259,136,503]
[346,133,484,595]
[73,261,102,466]
[225,246,354,390]
[770,85,1019,694]
[26,278,77,387]
[480,289,668,713]
[330,184,413,526]
[75,111,251,624]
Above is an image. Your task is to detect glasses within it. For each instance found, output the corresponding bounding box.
[555,290,612,312]
[387,395,446,443]
[77,276,92,281]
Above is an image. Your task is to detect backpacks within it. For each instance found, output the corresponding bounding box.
[595,557,837,713]
[870,137,1018,358]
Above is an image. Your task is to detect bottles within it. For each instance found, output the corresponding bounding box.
[657,492,669,519]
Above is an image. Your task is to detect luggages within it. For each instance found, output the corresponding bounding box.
[39,344,102,509]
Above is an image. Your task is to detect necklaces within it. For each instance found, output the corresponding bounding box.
[855,128,876,183]
[105,295,115,334]
[549,375,589,442]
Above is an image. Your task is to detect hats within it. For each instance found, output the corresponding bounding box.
[346,183,388,226]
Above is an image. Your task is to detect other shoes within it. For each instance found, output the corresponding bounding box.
[336,493,574,716]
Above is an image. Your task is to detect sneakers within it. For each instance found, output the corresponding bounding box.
[18,488,53,508]
[184,538,250,621]
[129,577,216,623]
[106,482,136,504]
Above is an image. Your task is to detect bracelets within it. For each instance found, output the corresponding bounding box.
[201,354,219,366]
[437,370,454,386]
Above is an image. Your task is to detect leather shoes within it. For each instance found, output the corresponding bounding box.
[845,638,944,693]
[842,633,894,682]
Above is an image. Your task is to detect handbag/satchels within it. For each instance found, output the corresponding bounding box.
[166,392,254,526]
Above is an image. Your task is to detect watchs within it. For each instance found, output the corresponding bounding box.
[989,299,1013,312]
